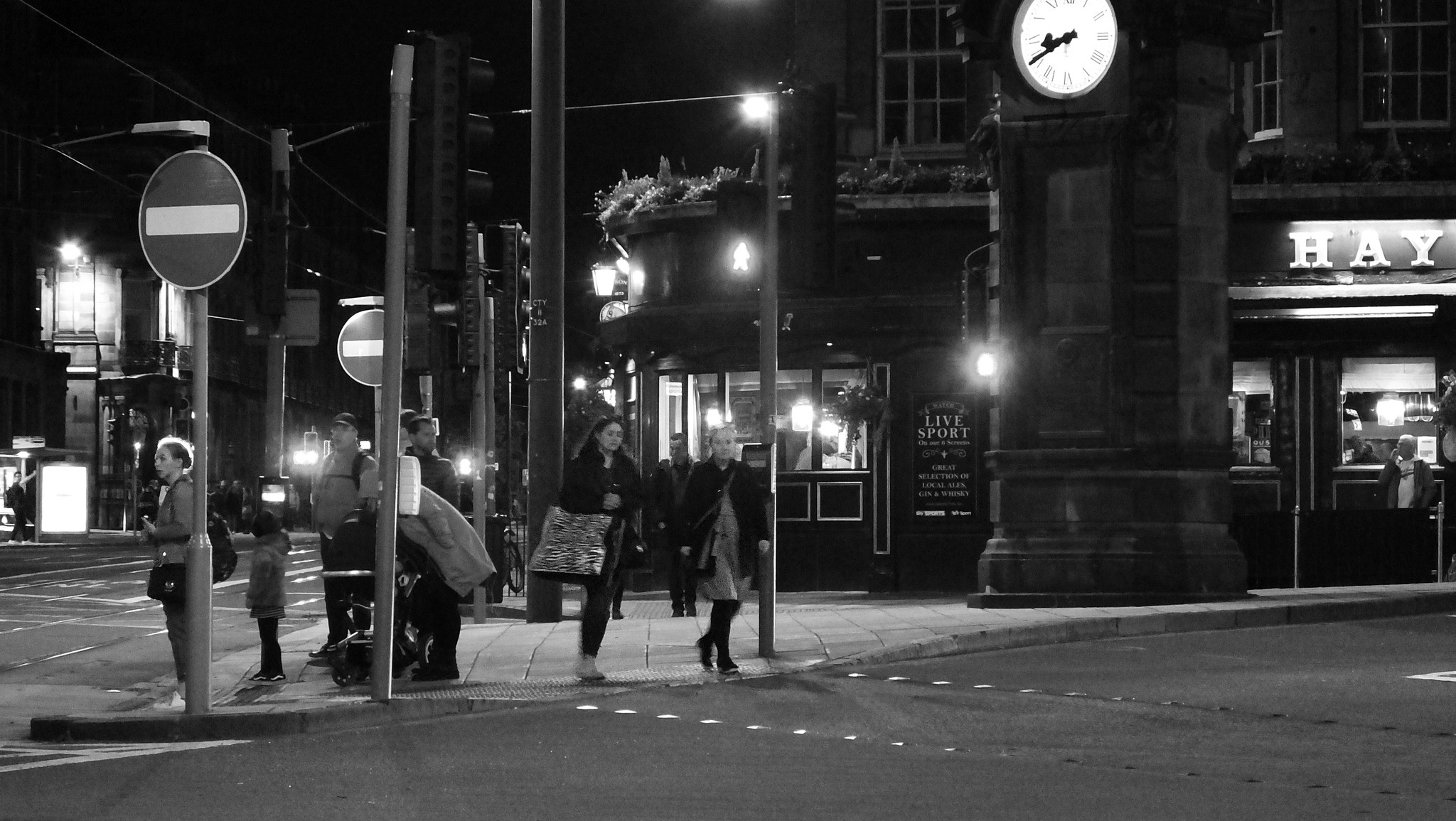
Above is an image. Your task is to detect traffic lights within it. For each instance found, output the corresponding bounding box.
[434,230,483,371]
[500,223,535,374]
[410,28,499,273]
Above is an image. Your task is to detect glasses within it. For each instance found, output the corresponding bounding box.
[1397,444,1411,448]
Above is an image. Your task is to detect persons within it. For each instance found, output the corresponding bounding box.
[139,479,302,530]
[309,413,496,682]
[7,470,35,544]
[560,419,643,680]
[1378,434,1435,509]
[678,425,769,673]
[653,434,701,617]
[142,442,195,710]
[246,510,294,681]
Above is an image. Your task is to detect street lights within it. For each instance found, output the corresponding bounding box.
[741,92,779,658]
[130,120,215,717]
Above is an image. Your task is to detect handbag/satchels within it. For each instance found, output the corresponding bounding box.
[147,563,187,603]
[528,492,621,587]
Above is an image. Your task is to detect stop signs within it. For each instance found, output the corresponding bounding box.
[136,149,249,291]
[340,311,389,387]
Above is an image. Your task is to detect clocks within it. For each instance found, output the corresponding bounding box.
[1011,0,1119,100]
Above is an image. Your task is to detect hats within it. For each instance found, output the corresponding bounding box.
[330,413,359,430]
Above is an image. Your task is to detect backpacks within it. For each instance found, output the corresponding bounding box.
[167,477,238,585]
[2,484,19,508]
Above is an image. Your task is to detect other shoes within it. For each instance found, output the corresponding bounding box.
[411,645,460,685]
[716,658,739,672]
[671,604,696,617]
[248,671,286,685]
[22,540,33,545]
[612,608,624,620]
[574,654,604,680]
[308,643,343,658]
[8,540,19,544]
[696,634,714,670]
[149,689,187,711]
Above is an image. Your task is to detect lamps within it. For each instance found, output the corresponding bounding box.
[590,242,618,298]
[792,376,813,432]
